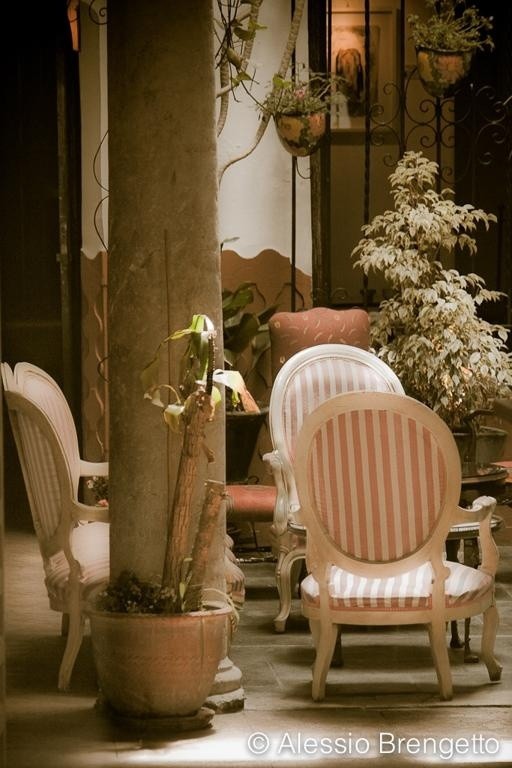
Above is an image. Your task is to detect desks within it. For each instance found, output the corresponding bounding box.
[274,515,504,633]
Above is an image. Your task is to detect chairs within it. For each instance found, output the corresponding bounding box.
[0,359,248,693]
[268,307,371,520]
[292,389,504,704]
[258,344,407,633]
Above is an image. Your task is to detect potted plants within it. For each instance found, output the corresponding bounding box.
[262,62,336,159]
[403,0,498,95]
[78,310,261,715]
